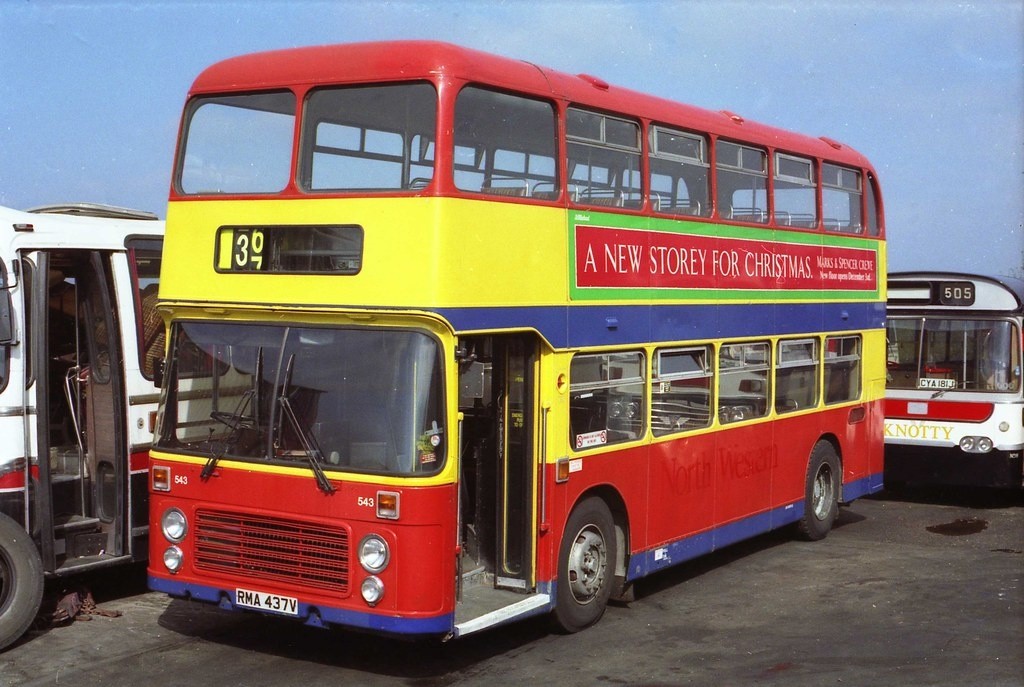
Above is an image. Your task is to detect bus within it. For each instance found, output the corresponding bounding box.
[883,268,1024,507]
[1,201,334,653]
[136,37,888,647]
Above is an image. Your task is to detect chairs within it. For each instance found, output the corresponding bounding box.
[823,219,861,234]
[409,178,433,190]
[758,397,808,415]
[660,198,701,216]
[480,177,530,197]
[624,191,661,211]
[791,217,841,232]
[733,207,764,223]
[700,201,734,220]
[774,213,816,229]
[578,187,625,208]
[733,210,792,226]
[531,182,579,203]
